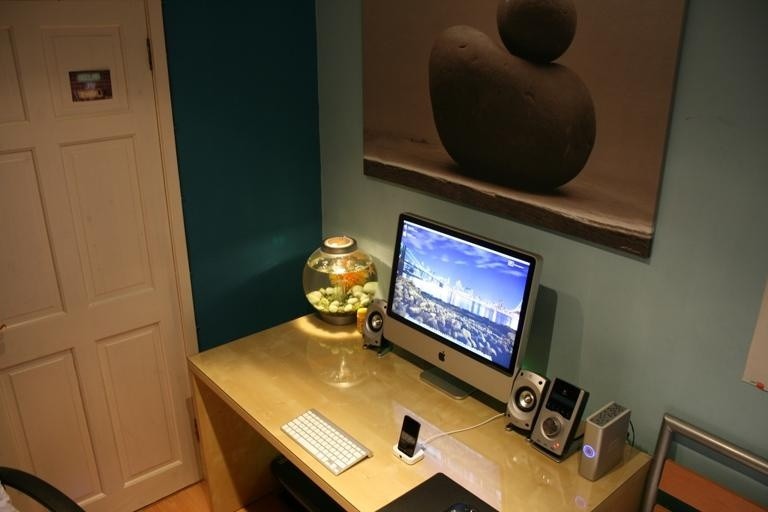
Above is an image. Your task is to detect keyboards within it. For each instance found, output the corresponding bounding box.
[281,409,372,475]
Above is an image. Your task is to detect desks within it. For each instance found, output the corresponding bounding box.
[186,309,654,511]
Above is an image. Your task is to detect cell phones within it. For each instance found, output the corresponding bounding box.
[397,415,422,457]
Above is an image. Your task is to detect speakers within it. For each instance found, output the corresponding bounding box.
[361,298,388,355]
[530,377,589,465]
[505,370,549,439]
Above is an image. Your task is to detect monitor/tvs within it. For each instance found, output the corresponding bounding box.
[382,211,542,401]
